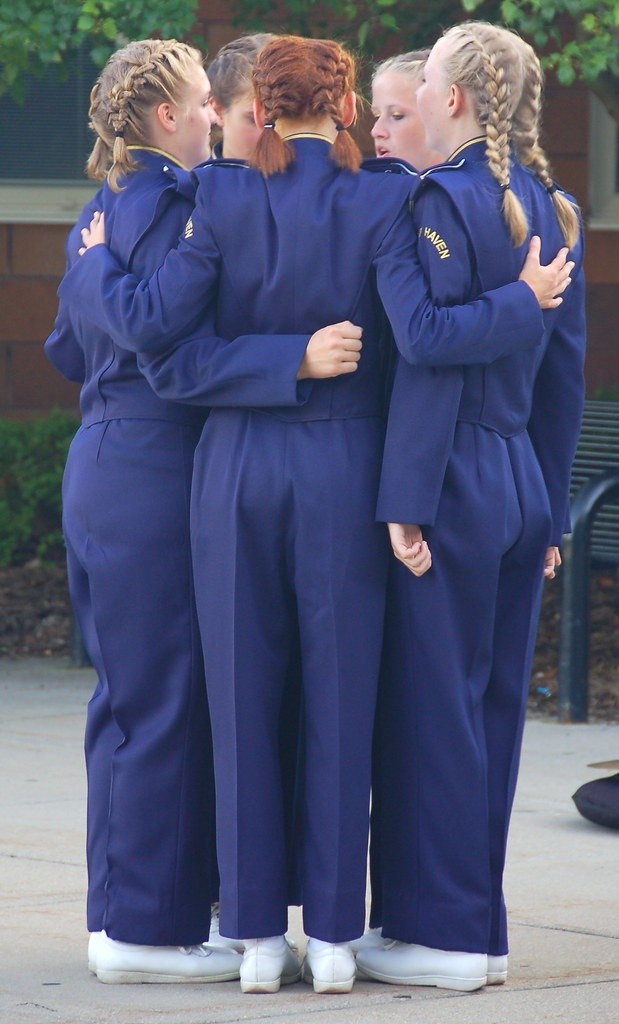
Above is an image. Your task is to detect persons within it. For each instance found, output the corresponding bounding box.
[351,23,587,992]
[44,33,575,994]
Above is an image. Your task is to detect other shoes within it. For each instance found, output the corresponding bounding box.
[355,937,488,992]
[484,953,509,986]
[303,936,357,994]
[87,930,102,975]
[202,913,300,955]
[93,933,246,985]
[239,932,302,993]
[347,926,396,954]
